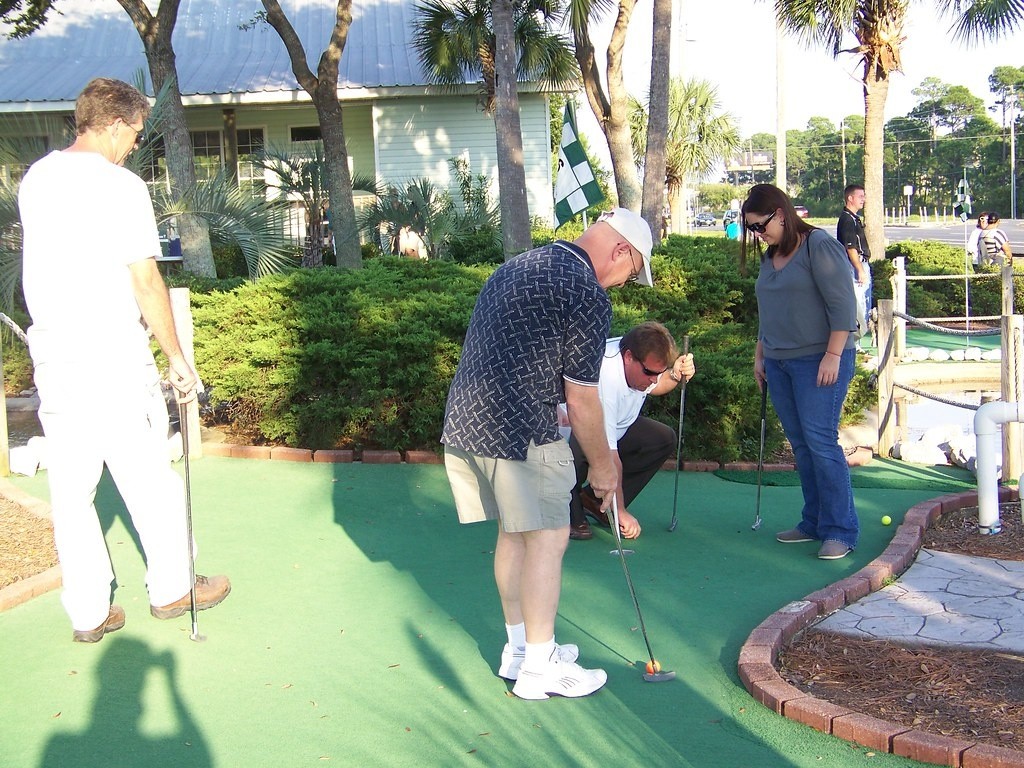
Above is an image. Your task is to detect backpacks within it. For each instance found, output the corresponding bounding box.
[977,228,998,270]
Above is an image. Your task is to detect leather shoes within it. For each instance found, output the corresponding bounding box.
[569,517,593,540]
[580,487,611,528]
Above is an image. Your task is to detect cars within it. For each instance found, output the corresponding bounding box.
[794,206,809,217]
[694,214,716,227]
[662,207,671,219]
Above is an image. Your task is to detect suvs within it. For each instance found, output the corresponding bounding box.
[723,210,739,231]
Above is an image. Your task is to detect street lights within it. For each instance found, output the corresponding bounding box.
[695,161,699,214]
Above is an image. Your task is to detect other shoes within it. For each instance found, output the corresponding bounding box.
[856,349,869,355]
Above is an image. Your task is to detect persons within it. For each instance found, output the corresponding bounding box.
[741,183,860,560]
[977,213,1010,276]
[1011,273,1013,275]
[726,219,740,240]
[18,78,231,644]
[557,320,696,540]
[441,207,653,700]
[836,185,872,338]
[968,212,988,274]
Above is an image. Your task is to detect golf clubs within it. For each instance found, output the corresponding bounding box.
[603,495,677,684]
[176,376,208,643]
[751,367,768,531]
[667,334,690,533]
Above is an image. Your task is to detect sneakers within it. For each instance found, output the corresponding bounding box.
[150,574,231,621]
[499,640,578,681]
[818,541,851,559]
[776,528,814,543]
[512,645,607,699]
[72,605,126,643]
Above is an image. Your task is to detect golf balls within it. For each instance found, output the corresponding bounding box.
[882,515,892,525]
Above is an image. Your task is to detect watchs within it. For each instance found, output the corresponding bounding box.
[669,369,689,385]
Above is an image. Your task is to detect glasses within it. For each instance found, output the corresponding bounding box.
[631,353,668,376]
[111,115,145,145]
[747,211,776,233]
[618,243,639,283]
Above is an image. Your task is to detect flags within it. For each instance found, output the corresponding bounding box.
[553,97,606,233]
[954,178,971,223]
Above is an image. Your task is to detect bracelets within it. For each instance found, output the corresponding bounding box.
[826,351,841,357]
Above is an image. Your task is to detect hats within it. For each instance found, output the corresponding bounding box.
[597,208,653,288]
